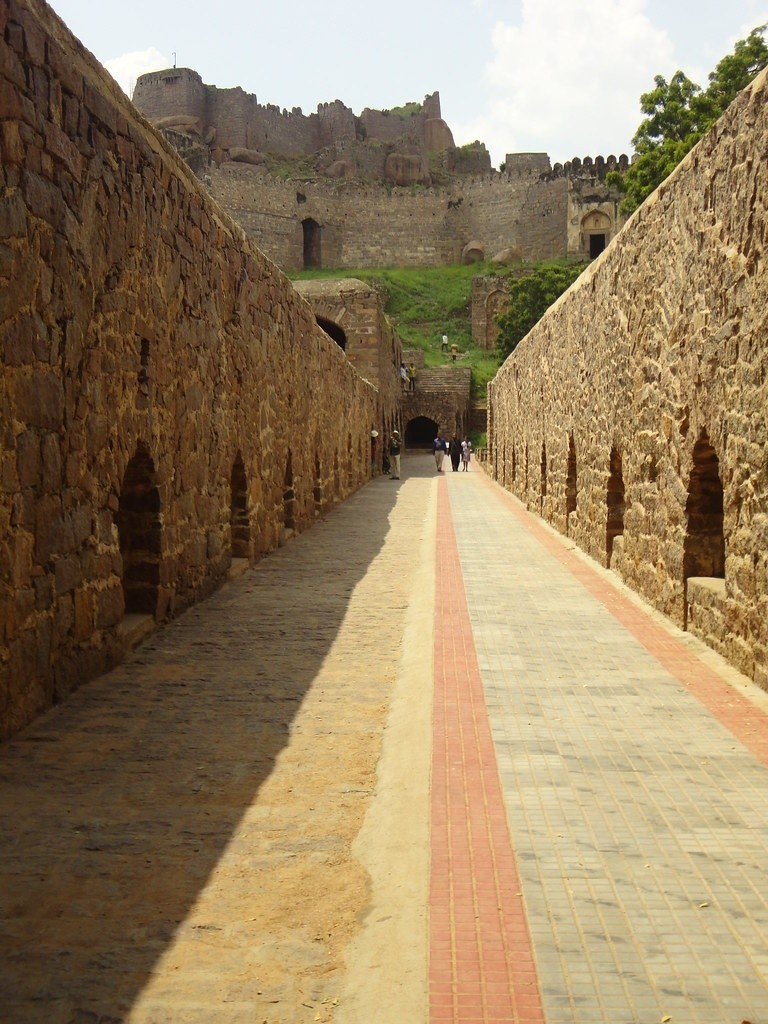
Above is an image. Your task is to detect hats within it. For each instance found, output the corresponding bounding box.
[371,430,378,437]
[393,429,400,436]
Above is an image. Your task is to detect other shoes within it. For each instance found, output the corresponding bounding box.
[388,475,400,480]
[466,468,468,472]
[437,467,442,472]
[462,468,465,472]
[452,466,458,472]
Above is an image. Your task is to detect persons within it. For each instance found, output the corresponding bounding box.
[433,432,446,472]
[383,430,401,480]
[409,363,416,392]
[370,430,378,464]
[442,334,448,352]
[461,435,472,472]
[448,433,462,472]
[401,363,409,391]
[451,347,457,364]
